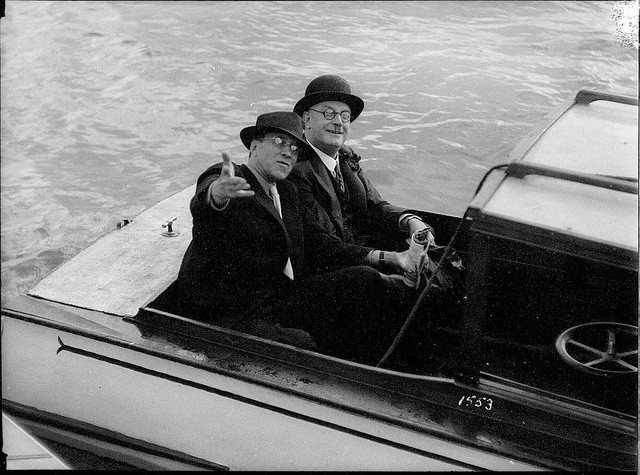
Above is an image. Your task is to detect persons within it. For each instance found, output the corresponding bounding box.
[293,74,437,288]
[177,111,381,355]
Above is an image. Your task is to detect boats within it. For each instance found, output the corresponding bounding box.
[2,85,639,474]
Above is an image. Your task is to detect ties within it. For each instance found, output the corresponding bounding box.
[270,186,294,281]
[335,161,346,192]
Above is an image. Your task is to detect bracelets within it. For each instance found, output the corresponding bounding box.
[406,215,422,225]
[378,251,386,266]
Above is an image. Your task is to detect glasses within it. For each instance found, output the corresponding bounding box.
[310,108,352,122]
[257,136,303,154]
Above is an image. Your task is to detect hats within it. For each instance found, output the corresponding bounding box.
[240,112,309,163]
[294,75,364,129]
